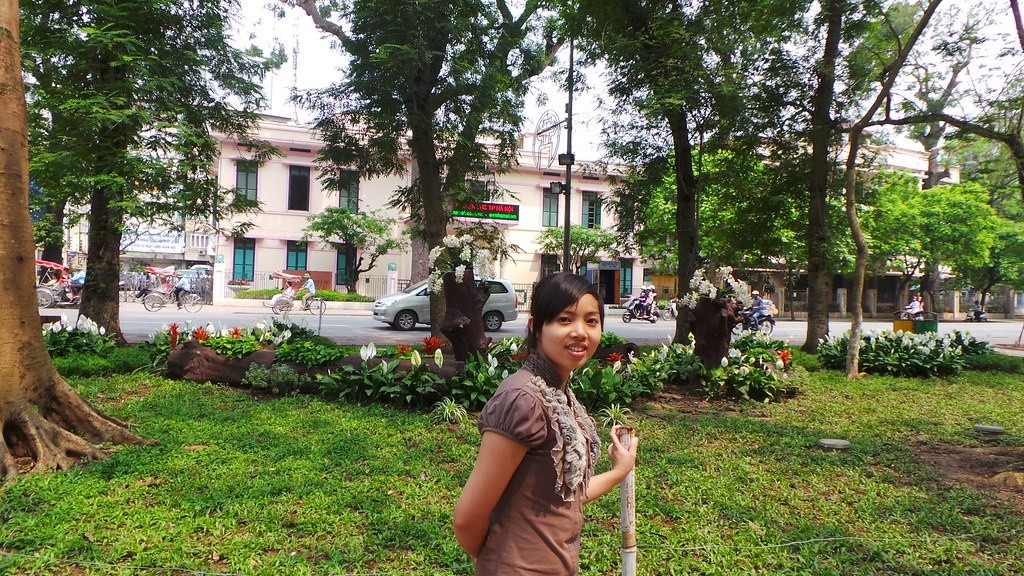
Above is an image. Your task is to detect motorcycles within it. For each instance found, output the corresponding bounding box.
[731,309,776,337]
[900,306,924,322]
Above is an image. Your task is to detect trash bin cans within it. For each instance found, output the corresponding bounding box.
[913,310,938,335]
[892,311,915,334]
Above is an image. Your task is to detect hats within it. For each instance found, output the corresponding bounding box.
[303,273,311,278]
[176,273,183,278]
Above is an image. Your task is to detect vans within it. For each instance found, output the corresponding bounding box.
[373,276,518,332]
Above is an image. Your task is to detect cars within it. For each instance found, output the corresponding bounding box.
[72,272,85,286]
[174,270,204,296]
[119,272,138,290]
[190,265,213,293]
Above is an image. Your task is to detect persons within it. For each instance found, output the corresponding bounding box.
[905,296,924,313]
[271,280,293,307]
[449,271,638,575]
[175,273,191,309]
[44,273,60,286]
[300,273,316,309]
[971,301,983,319]
[69,264,86,300]
[744,290,764,330]
[133,278,167,301]
[635,284,658,320]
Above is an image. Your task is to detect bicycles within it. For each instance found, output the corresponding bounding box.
[62,280,84,309]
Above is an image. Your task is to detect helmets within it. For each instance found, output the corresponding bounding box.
[640,286,646,290]
[650,285,655,289]
[646,286,652,290]
[752,290,759,295]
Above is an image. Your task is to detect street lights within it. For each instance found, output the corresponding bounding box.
[532,28,575,271]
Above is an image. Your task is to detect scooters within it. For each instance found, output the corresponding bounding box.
[662,298,678,321]
[621,297,660,323]
[965,308,987,322]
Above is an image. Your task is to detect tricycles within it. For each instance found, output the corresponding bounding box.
[143,266,203,313]
[262,272,326,315]
[35,260,69,309]
